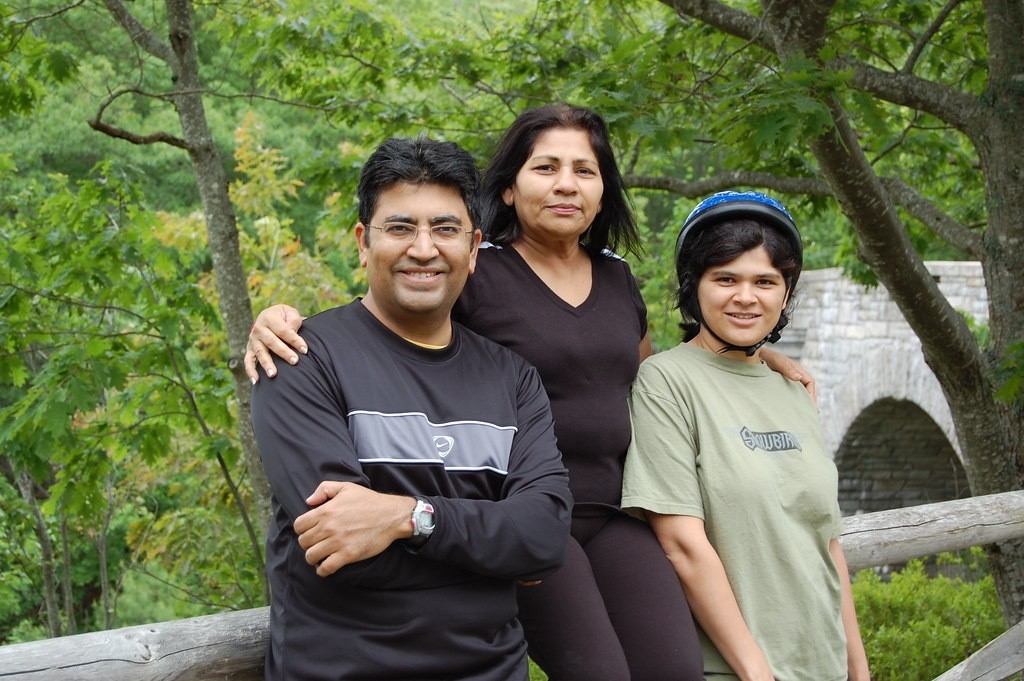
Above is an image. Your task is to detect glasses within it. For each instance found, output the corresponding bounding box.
[365,222,474,244]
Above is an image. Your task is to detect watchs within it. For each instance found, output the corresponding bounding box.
[411,496,435,543]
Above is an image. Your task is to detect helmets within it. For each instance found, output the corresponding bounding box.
[674,190,804,270]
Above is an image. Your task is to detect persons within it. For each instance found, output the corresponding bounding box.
[622,190,869,681]
[243,105,704,681]
[251,138,573,681]
